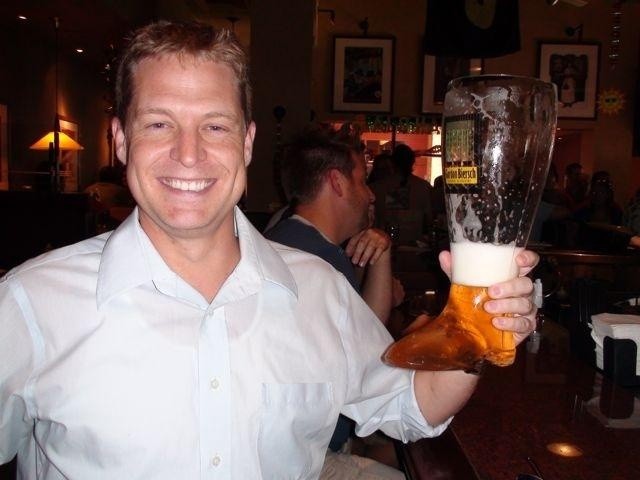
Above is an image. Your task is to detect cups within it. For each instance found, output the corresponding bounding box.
[381,74,559,372]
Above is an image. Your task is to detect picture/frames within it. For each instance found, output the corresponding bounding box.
[329,35,603,122]
[54,113,82,193]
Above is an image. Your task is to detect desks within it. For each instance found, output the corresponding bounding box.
[395,269,640,479]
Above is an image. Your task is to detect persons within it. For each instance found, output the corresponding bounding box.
[82,165,130,206]
[0,14,540,480]
[259,121,640,480]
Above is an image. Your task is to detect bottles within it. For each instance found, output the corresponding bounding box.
[526,278,545,357]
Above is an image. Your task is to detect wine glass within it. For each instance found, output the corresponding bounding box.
[362,112,439,134]
